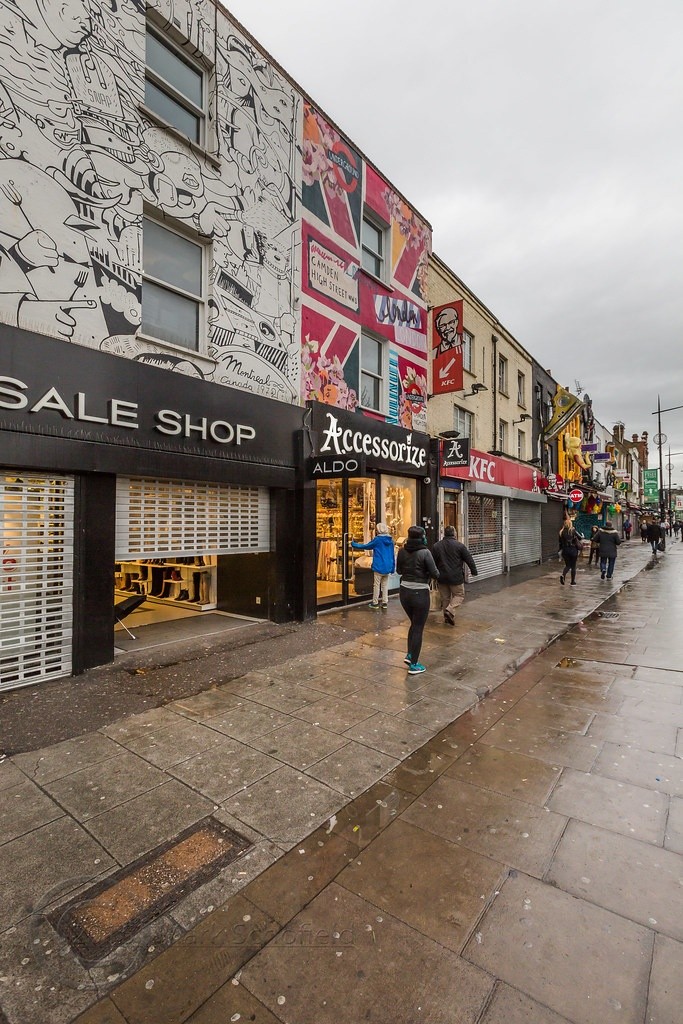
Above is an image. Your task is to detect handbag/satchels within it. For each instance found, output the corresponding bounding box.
[558,549,563,562]
[591,541,598,548]
[626,528,631,532]
[429,579,442,612]
[572,529,583,550]
[658,538,665,551]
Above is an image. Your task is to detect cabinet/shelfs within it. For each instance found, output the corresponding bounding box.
[114,562,217,611]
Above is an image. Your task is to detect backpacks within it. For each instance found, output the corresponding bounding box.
[641,524,647,530]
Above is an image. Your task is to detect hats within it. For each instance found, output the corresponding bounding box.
[377,523,389,534]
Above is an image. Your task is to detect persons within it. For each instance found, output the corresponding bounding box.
[639,519,683,554]
[351,523,395,609]
[587,525,599,565]
[432,525,478,626]
[396,526,440,675]
[591,520,621,581]
[558,520,582,585]
[623,520,632,540]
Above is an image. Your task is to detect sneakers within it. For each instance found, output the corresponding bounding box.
[408,662,426,674]
[380,603,387,608]
[404,653,411,666]
[369,603,379,609]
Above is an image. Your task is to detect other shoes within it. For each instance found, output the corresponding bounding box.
[601,569,606,579]
[588,562,591,565]
[607,577,612,579]
[595,563,598,565]
[570,582,577,586]
[444,611,455,626]
[652,550,656,554]
[560,575,565,585]
[445,618,449,623]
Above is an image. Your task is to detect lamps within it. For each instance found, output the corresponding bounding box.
[527,457,541,464]
[464,383,488,397]
[513,414,533,426]
[439,431,460,439]
[486,450,504,456]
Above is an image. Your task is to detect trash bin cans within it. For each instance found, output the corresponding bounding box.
[353,564,374,595]
